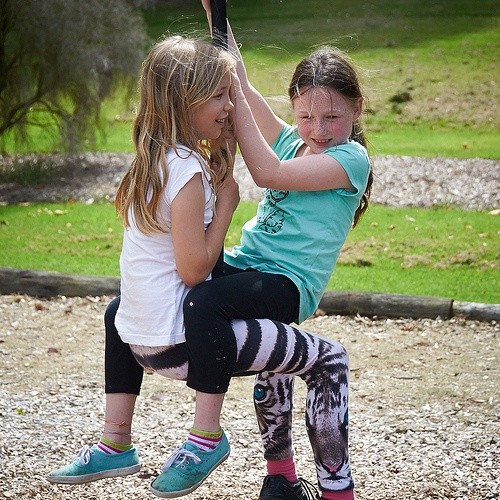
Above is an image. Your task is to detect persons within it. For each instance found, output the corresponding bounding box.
[113,27,356,500]
[45,1,376,499]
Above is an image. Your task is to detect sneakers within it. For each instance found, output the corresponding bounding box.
[148,427,231,497]
[254,472,318,500]
[47,442,142,484]
